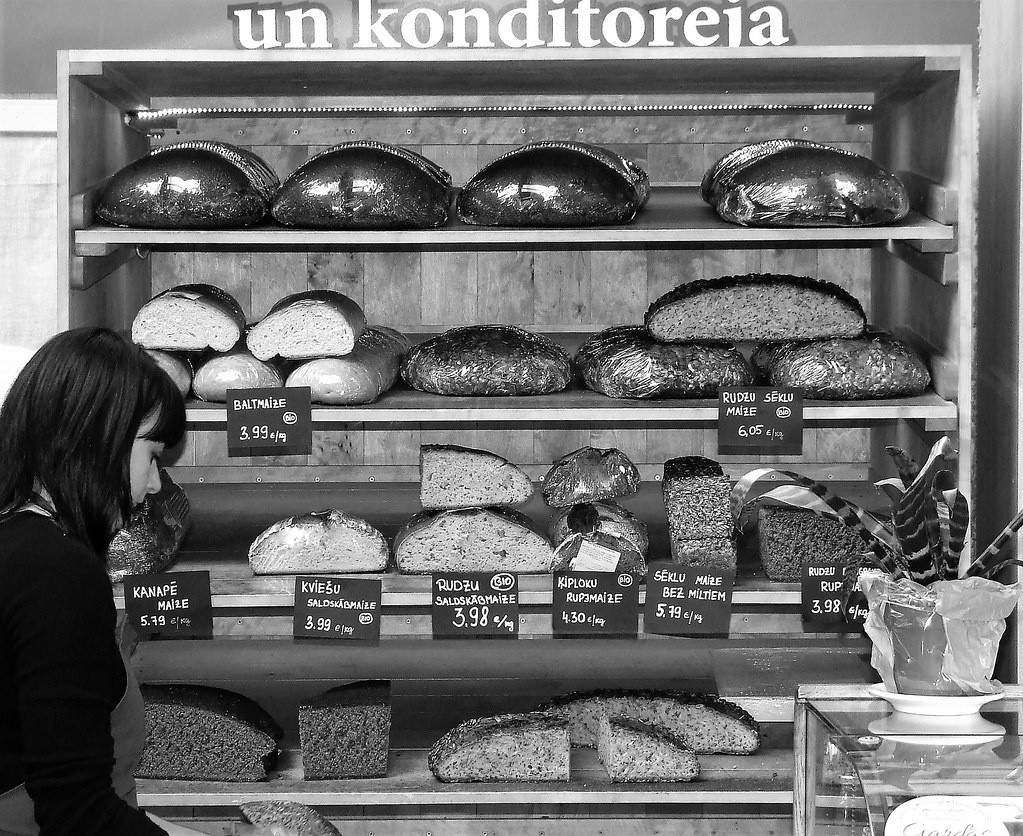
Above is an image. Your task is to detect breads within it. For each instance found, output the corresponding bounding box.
[94,140,931,836]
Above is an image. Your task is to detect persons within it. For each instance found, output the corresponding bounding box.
[0,326,187,836]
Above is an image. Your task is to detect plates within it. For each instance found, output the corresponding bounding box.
[868,712,1006,746]
[868,683,1006,716]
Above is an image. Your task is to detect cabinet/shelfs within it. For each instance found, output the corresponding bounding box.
[794,682,1023,836]
[55,45,980,836]
